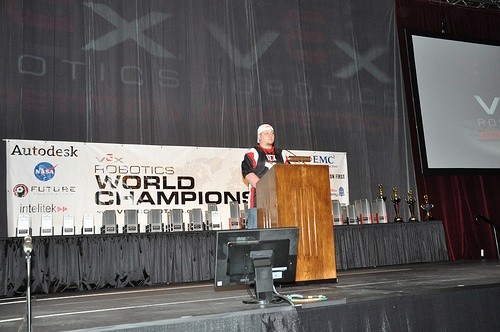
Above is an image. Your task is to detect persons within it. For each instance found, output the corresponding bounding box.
[242,124,291,228]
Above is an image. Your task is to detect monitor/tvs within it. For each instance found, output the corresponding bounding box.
[214,226,300,304]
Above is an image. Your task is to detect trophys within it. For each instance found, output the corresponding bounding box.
[420,195,434,223]
[390,186,404,223]
[376,184,387,202]
[405,190,418,222]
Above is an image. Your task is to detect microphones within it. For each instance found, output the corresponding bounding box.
[285,148,306,165]
[23,236,33,253]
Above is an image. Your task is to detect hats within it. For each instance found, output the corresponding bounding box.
[257,124,274,144]
